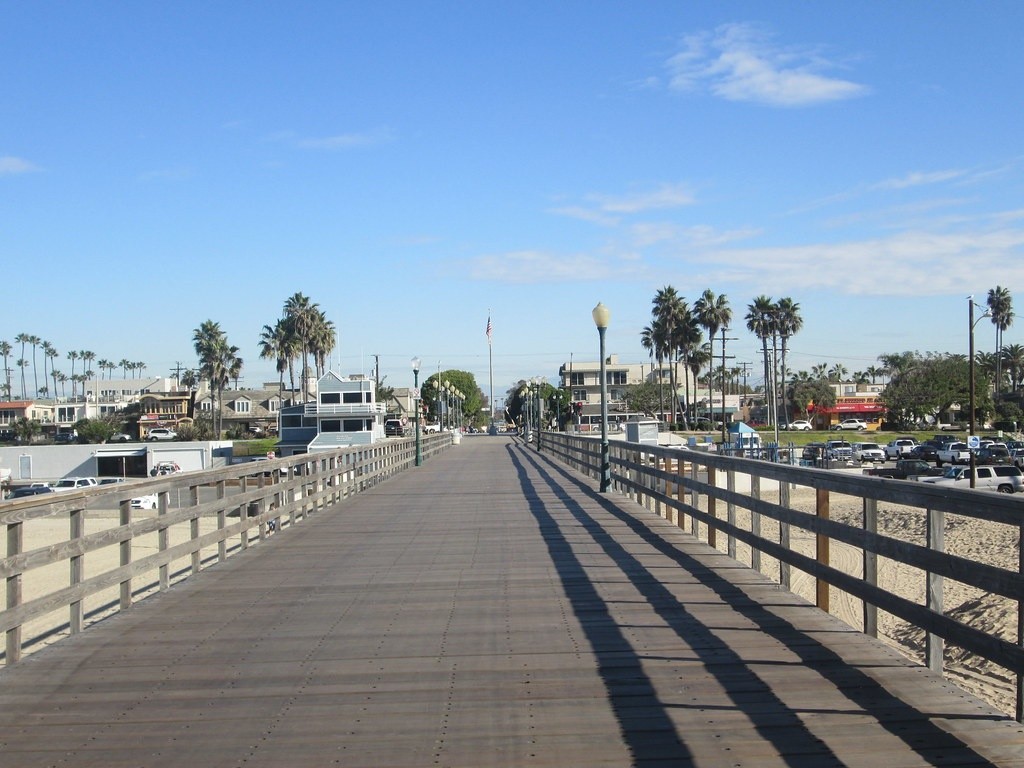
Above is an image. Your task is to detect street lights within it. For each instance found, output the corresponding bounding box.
[450,384,455,429]
[455,388,459,428]
[968,312,992,488]
[411,356,421,464]
[432,379,439,432]
[590,301,611,491]
[444,380,450,430]
[519,374,548,451]
[459,392,463,427]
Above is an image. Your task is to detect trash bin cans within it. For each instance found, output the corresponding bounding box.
[451,433,461,445]
[527,431,534,442]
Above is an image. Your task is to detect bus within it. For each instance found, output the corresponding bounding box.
[575,412,690,429]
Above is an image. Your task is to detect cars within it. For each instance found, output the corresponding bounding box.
[779,419,813,431]
[746,419,768,429]
[833,417,868,431]
[659,431,1024,495]
[51,432,77,444]
[5,458,182,511]
[110,431,132,443]
[421,421,507,435]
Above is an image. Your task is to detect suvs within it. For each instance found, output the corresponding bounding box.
[689,417,723,431]
[385,418,406,438]
[147,427,178,443]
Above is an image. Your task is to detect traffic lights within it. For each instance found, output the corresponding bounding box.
[576,401,583,410]
